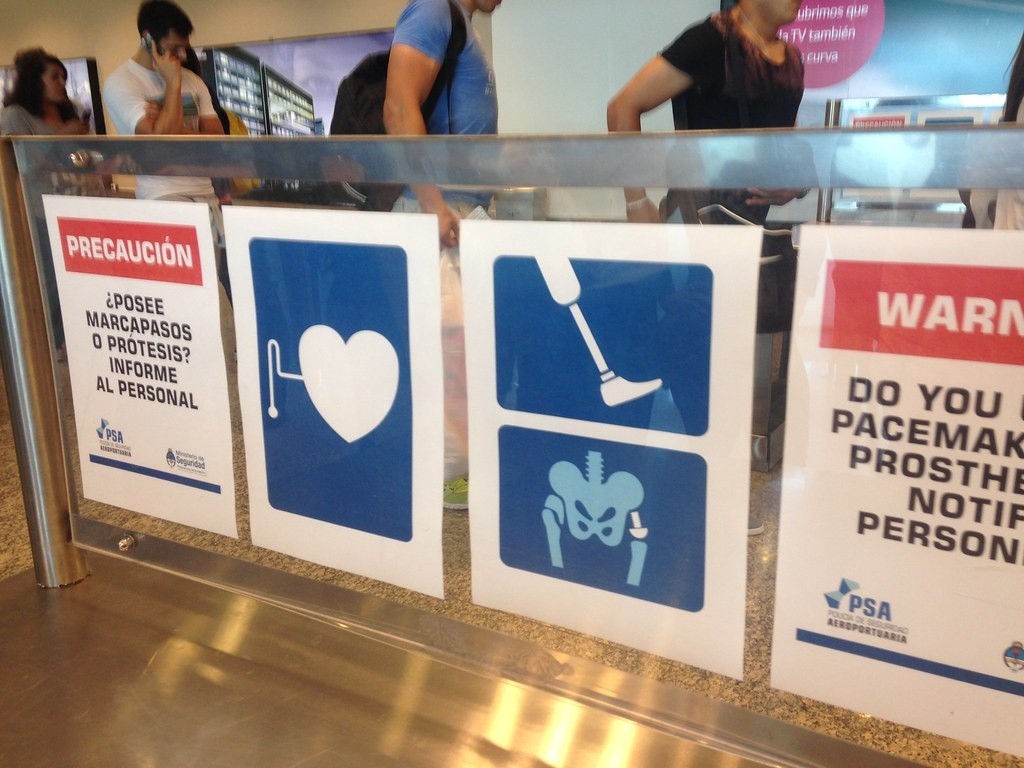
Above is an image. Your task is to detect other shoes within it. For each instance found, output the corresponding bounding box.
[748,516,764,535]
[443,478,468,510]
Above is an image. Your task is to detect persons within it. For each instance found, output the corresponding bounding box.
[992,33,1024,232]
[181,45,260,308]
[0,45,115,367]
[101,1,226,282]
[366,0,501,510]
[606,0,815,329]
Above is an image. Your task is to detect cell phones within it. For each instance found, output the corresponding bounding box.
[141,32,164,58]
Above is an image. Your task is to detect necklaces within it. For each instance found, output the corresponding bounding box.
[736,5,768,56]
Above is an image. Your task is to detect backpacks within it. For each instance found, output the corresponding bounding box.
[221,107,261,194]
[328,0,468,212]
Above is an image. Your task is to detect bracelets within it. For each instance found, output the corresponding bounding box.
[625,197,649,210]
[796,188,808,199]
